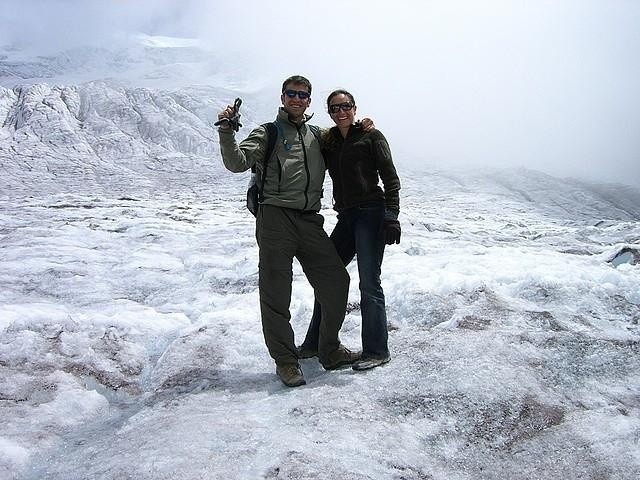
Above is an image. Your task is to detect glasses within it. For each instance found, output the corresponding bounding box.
[328,102,354,113]
[282,90,310,99]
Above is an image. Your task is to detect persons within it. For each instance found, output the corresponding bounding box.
[219,74,375,387]
[296,89,401,371]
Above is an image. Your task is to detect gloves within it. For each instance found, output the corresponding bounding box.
[214,96,244,133]
[381,207,402,245]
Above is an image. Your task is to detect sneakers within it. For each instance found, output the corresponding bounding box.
[319,343,391,373]
[295,344,319,360]
[275,361,307,388]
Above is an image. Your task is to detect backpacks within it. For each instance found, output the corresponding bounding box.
[246,124,278,218]
